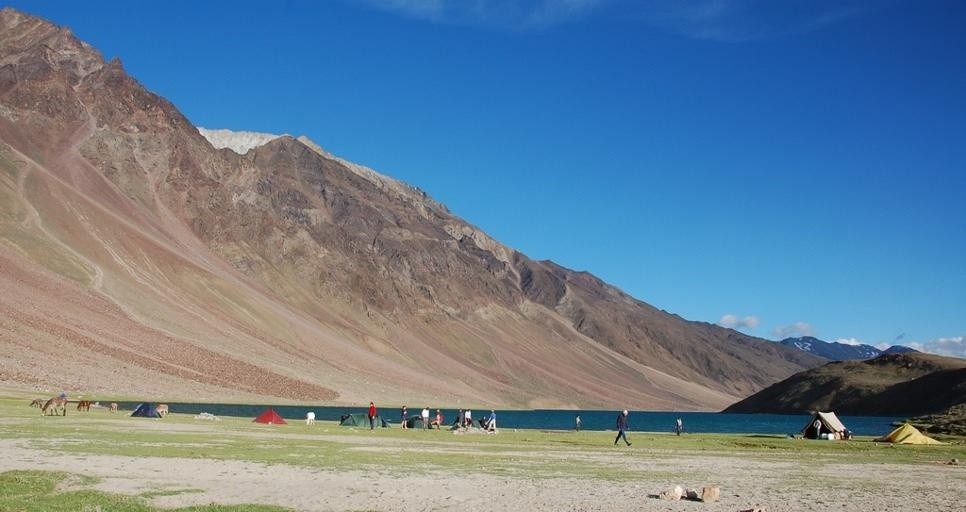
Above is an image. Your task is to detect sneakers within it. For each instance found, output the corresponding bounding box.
[614,444,618,445]
[627,443,632,446]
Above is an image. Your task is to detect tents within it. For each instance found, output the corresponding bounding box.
[130,402,161,418]
[401,415,433,429]
[251,406,287,425]
[339,412,391,427]
[449,416,482,431]
[873,423,949,445]
[802,412,846,440]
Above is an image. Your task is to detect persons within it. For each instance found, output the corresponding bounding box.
[401,406,408,429]
[575,415,582,432]
[436,408,441,432]
[457,408,497,435]
[421,406,429,430]
[614,409,632,446]
[367,402,376,431]
